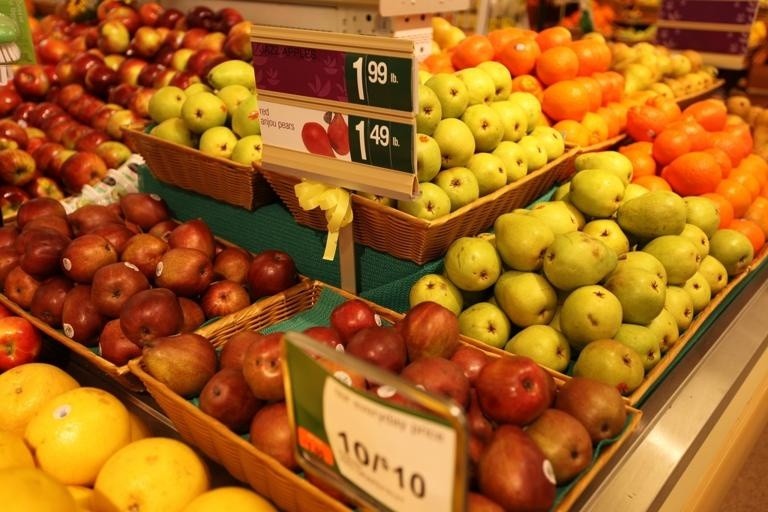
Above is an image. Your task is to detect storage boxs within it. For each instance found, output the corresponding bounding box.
[656,0,760,55]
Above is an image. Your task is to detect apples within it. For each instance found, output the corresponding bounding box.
[0,0,626,512]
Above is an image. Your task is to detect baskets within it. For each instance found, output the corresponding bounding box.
[0,218,316,393]
[579,80,728,156]
[128,279,642,512]
[261,143,579,266]
[401,241,766,405]
[120,125,277,211]
[0,78,767,512]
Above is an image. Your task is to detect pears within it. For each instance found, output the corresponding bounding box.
[147,15,754,398]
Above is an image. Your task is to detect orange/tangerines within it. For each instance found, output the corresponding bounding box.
[419,24,768,256]
